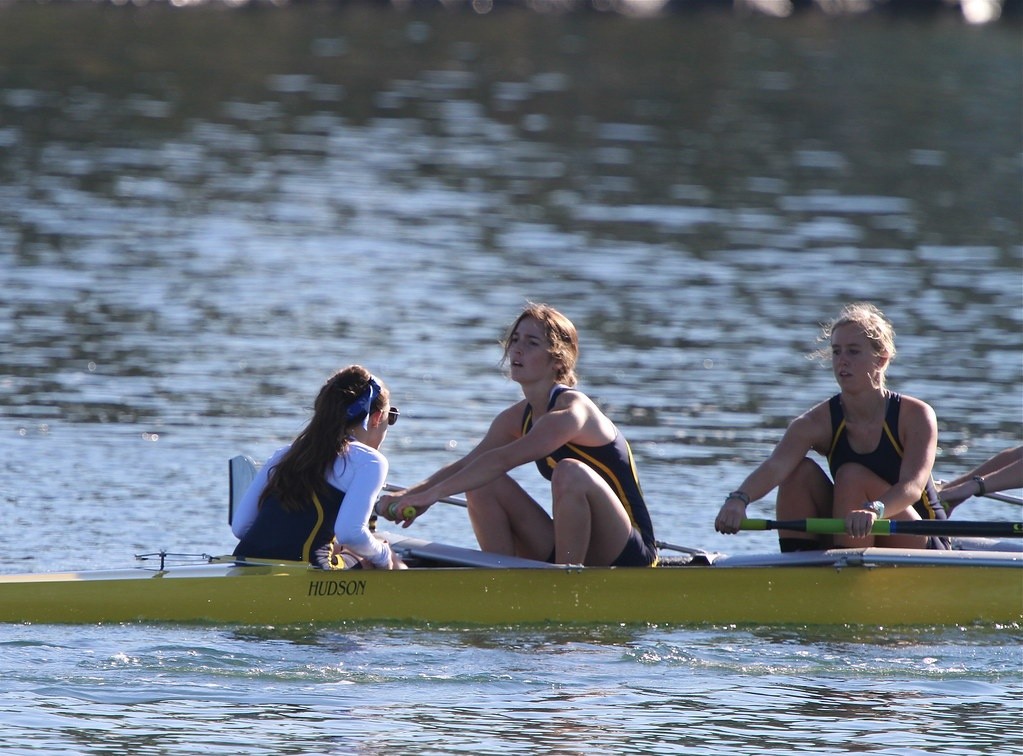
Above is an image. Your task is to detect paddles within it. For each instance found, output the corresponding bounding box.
[241,458,420,518]
[725,511,1023,540]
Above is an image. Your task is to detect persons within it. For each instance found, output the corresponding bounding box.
[937,444,1023,516]
[231,364,409,569]
[714,303,955,553]
[378,301,659,567]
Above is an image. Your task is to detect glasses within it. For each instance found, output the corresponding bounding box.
[373,406,400,425]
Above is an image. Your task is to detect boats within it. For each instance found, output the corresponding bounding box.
[0,451,1022,637]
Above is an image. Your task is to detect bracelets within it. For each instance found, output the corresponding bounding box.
[864,500,884,519]
[725,491,749,505]
[973,476,985,497]
